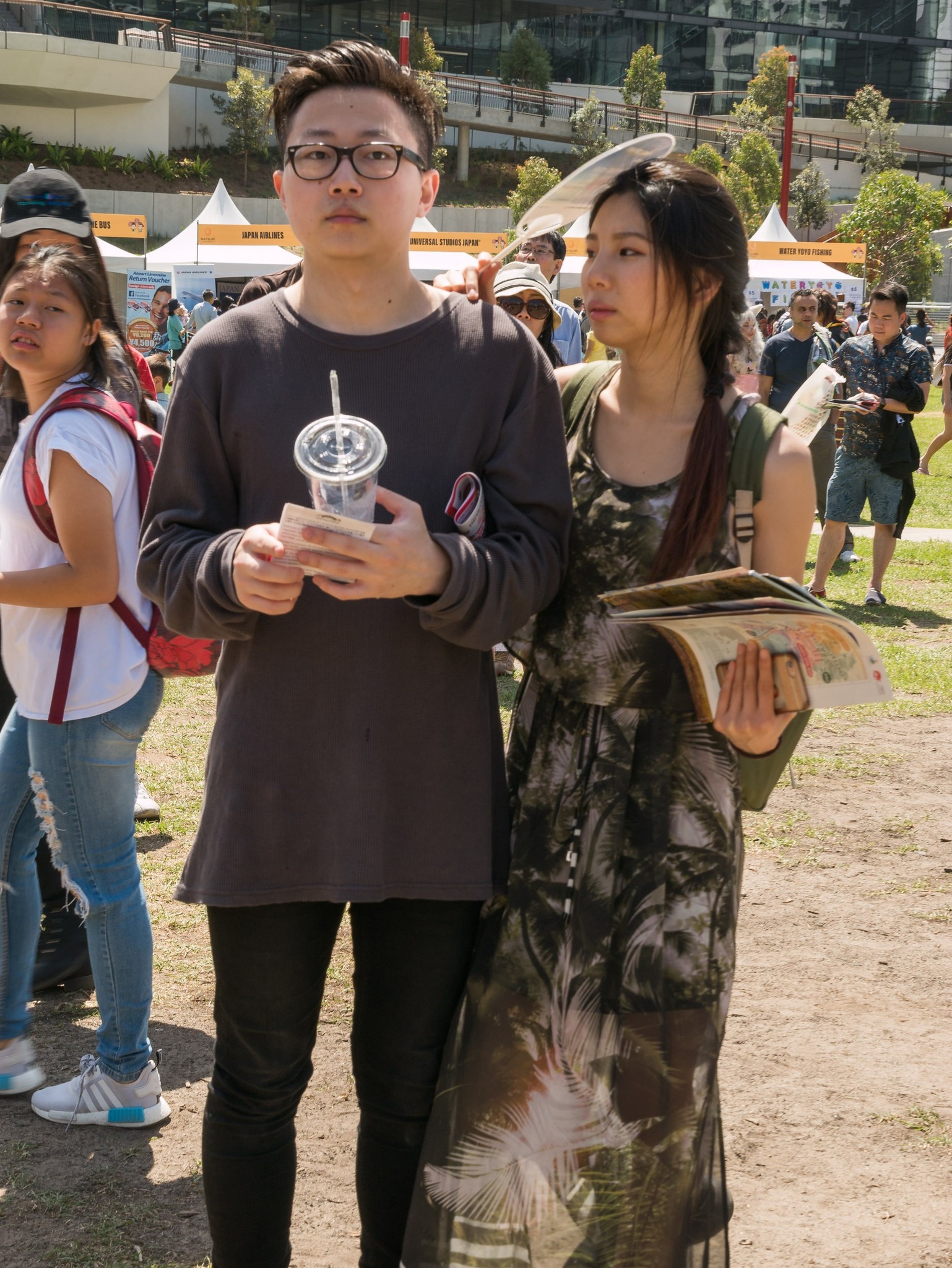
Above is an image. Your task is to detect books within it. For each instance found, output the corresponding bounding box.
[444,472,487,540]
[593,567,891,722]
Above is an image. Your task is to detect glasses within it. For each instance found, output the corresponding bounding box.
[282,142,427,180]
[519,244,559,258]
[843,307,850,310]
[499,294,550,320]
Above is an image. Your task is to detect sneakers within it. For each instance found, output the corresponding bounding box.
[30,1054,172,1134]
[0,1034,47,1095]
[130,773,161,818]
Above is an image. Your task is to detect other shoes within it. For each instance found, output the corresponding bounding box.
[27,898,96,994]
[802,585,827,600]
[840,550,860,563]
[865,589,887,605]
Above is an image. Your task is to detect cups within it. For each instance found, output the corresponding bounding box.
[293,414,388,525]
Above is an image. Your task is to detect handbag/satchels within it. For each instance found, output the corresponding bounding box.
[931,356,944,389]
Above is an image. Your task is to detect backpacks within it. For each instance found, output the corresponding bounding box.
[24,386,220,724]
[560,360,814,810]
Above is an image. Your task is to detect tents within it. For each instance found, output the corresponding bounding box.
[93,176,865,414]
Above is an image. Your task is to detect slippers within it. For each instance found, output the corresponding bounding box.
[916,468,933,477]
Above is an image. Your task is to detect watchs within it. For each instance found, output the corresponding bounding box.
[878,397,886,410]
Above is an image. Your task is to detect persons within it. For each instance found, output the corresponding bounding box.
[0,166,143,991]
[384,163,817,1268]
[0,244,175,1128]
[143,285,173,357]
[146,230,952,604]
[135,41,581,1268]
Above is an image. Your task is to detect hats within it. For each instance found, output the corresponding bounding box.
[925,336,933,342]
[748,304,763,316]
[0,166,94,238]
[755,299,765,308]
[496,261,562,333]
[179,305,188,314]
[168,298,183,310]
